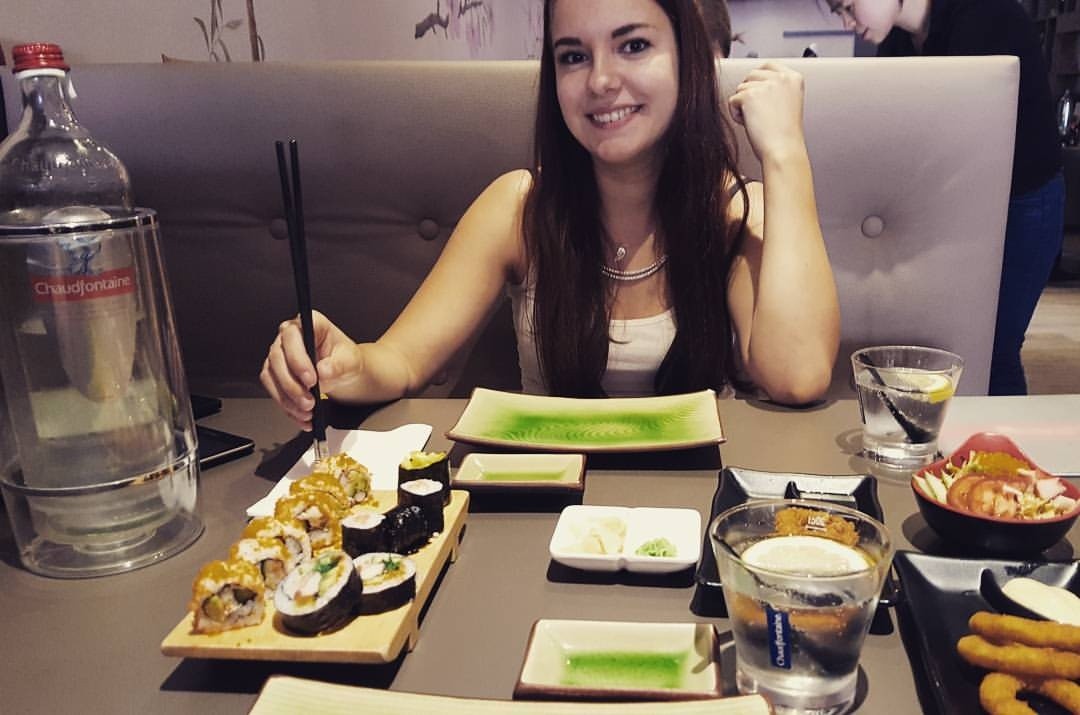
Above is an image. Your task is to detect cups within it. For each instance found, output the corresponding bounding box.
[708,498,895,715]
[0,210,204,583]
[851,345,965,477]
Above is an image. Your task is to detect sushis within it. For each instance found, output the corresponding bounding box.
[188,450,453,635]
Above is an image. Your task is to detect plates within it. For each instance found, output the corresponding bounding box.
[511,616,724,698]
[444,386,728,452]
[694,465,897,605]
[157,490,471,663]
[893,549,1080,714]
[450,453,587,495]
[549,504,701,573]
[244,673,774,715]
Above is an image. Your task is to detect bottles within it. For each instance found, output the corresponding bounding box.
[0,38,184,545]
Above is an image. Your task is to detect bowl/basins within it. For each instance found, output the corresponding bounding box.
[910,431,1079,558]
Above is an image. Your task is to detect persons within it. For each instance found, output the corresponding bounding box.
[257,1,846,435]
[828,1,1069,397]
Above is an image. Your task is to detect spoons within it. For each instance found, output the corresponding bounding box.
[858,351,935,443]
[711,533,855,679]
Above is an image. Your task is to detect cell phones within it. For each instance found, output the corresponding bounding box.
[195,424,255,472]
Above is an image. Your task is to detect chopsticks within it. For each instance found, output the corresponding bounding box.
[275,139,331,466]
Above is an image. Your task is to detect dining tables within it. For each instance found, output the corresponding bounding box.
[5,389,1078,715]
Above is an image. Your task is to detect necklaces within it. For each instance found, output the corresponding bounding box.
[599,254,668,281]
[602,228,652,263]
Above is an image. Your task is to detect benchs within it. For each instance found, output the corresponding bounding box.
[0,53,1025,400]
